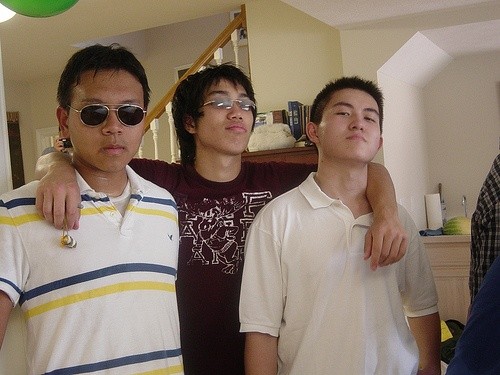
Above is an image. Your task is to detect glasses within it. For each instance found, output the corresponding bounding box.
[204,95,256,111]
[68,103,147,128]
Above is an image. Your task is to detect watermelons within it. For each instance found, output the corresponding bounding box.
[442,217,471,234]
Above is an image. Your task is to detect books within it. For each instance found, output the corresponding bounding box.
[288,101,313,142]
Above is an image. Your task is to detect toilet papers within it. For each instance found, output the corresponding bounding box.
[424,192,443,231]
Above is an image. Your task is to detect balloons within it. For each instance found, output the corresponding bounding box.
[0,0,79,18]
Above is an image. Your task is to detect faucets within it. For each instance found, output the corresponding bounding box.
[461,194,470,215]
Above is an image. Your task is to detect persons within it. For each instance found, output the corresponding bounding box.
[239,75,443,375]
[0,43,185,375]
[34,61,408,375]
